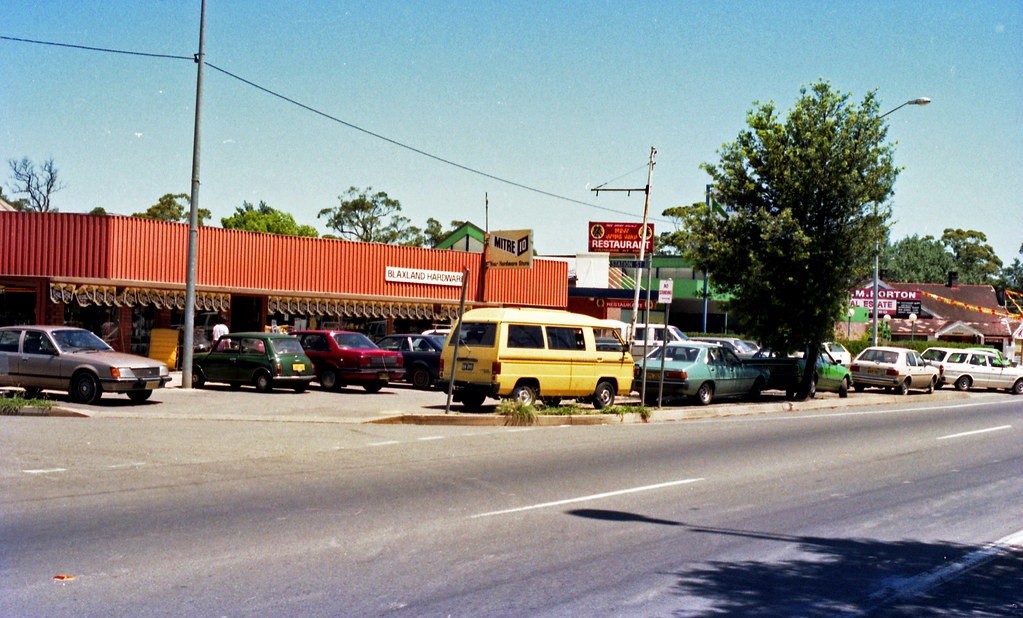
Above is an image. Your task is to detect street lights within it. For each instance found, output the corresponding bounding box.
[883,314,891,343]
[874,97,931,344]
[847,308,855,337]
[909,313,918,342]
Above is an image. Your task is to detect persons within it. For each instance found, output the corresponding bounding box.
[213,318,231,351]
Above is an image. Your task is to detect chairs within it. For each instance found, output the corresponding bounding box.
[26,338,42,353]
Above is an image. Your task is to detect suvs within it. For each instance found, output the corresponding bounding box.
[412,324,452,349]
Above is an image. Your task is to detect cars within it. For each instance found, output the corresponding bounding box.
[920,348,1023,395]
[287,329,404,393]
[957,347,1011,366]
[191,332,317,393]
[751,345,852,398]
[689,337,776,359]
[374,334,461,390]
[365,321,386,340]
[0,325,173,405]
[820,342,852,370]
[195,312,220,333]
[850,346,940,396]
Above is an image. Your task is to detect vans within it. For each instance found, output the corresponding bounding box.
[440,307,635,409]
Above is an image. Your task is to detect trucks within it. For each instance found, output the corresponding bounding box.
[602,320,689,360]
[634,341,771,406]
